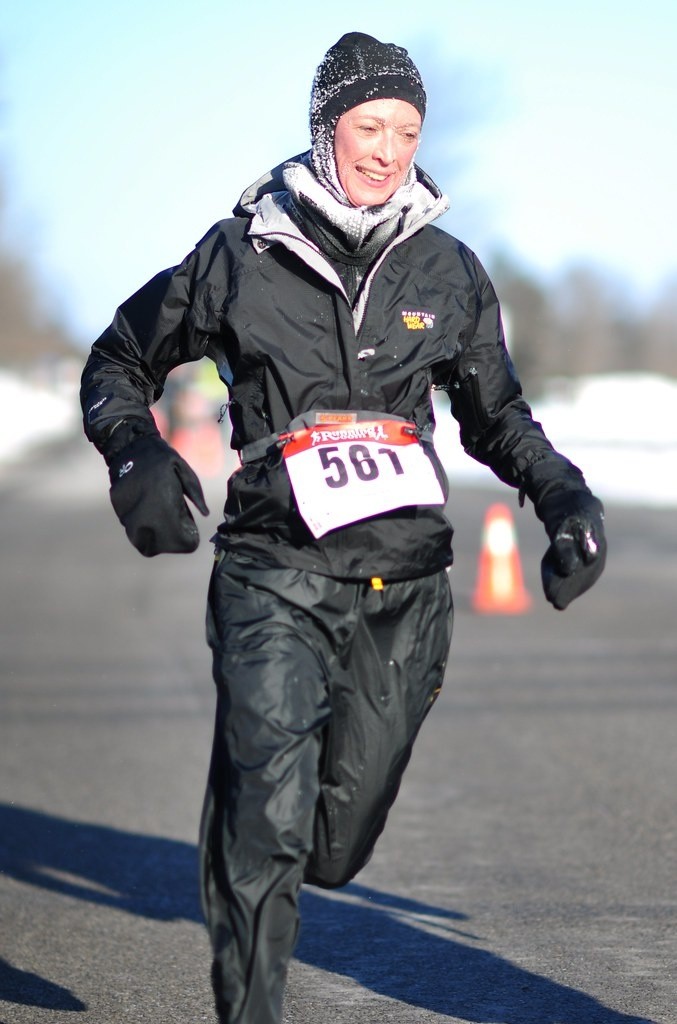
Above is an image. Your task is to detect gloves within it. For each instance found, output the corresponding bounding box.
[106,433,209,557]
[535,491,608,611]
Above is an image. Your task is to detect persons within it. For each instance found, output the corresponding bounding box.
[80,32,608,1024]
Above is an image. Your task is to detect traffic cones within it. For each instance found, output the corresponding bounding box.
[471,503,533,618]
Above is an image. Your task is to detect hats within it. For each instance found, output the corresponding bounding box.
[309,32,426,207]
